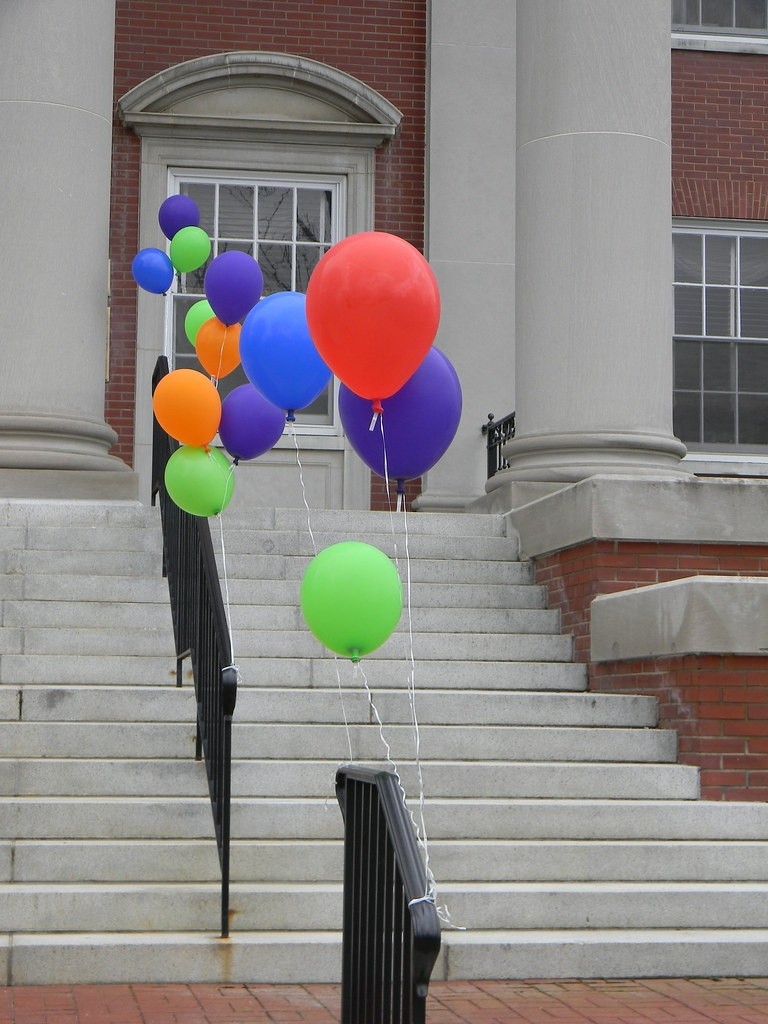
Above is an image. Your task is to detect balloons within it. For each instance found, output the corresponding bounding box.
[239,291,334,421]
[196,318,242,381]
[153,369,222,452]
[306,231,440,413]
[185,299,216,347]
[301,542,403,662]
[338,346,462,494]
[164,444,234,517]
[217,384,286,466]
[158,195,199,241]
[170,226,211,276]
[132,248,174,296]
[204,251,263,327]
